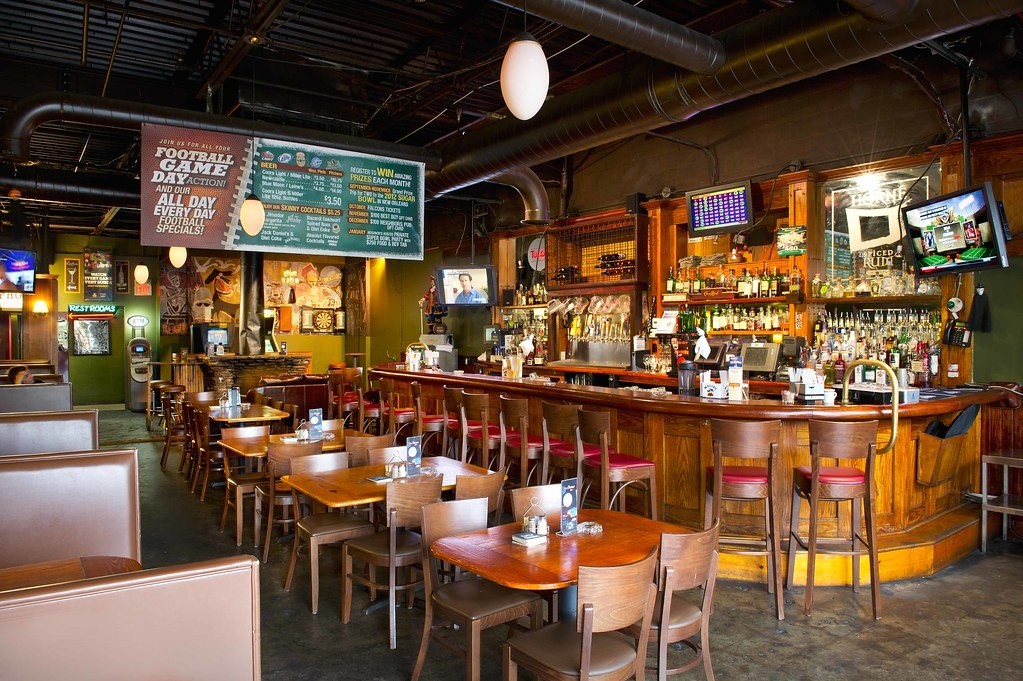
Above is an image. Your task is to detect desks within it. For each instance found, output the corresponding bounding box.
[216,429,376,544]
[0,555,142,600]
[206,402,290,488]
[280,455,508,617]
[429,509,696,624]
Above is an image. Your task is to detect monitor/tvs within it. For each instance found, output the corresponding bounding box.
[693,344,726,365]
[207,328,229,345]
[739,342,781,372]
[436,266,498,306]
[902,182,1009,279]
[0,248,37,294]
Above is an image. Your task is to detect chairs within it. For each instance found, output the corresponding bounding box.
[160,389,721,681]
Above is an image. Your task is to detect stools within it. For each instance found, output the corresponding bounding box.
[786,420,881,620]
[326,365,662,519]
[707,416,785,620]
[150,380,186,426]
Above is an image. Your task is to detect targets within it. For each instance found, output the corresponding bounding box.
[313,311,333,330]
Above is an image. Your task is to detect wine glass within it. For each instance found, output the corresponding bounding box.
[643,353,671,375]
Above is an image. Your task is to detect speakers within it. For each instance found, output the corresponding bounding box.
[625,192,646,213]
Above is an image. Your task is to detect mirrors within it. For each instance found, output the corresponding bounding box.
[814,159,943,287]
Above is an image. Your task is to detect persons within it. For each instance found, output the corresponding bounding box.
[7,365,45,385]
[418,275,442,333]
[455,274,486,303]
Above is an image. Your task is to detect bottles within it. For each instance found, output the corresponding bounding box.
[512,280,547,306]
[676,304,790,334]
[494,310,549,365]
[812,266,942,298]
[295,429,308,441]
[216,342,224,355]
[550,253,636,283]
[391,463,405,480]
[527,514,548,536]
[799,308,939,391]
[667,266,802,298]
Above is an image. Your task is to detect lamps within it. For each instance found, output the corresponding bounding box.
[32,300,49,317]
[500,0,550,121]
[169,246,187,268]
[240,49,265,237]
[134,246,149,285]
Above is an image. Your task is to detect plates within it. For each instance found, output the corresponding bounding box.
[454,370,464,375]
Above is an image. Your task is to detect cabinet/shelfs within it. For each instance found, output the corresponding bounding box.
[982,448,1023,553]
[489,146,974,400]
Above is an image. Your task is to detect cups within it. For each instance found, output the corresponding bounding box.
[781,390,794,404]
[546,295,630,315]
[678,360,697,401]
[172,347,189,364]
